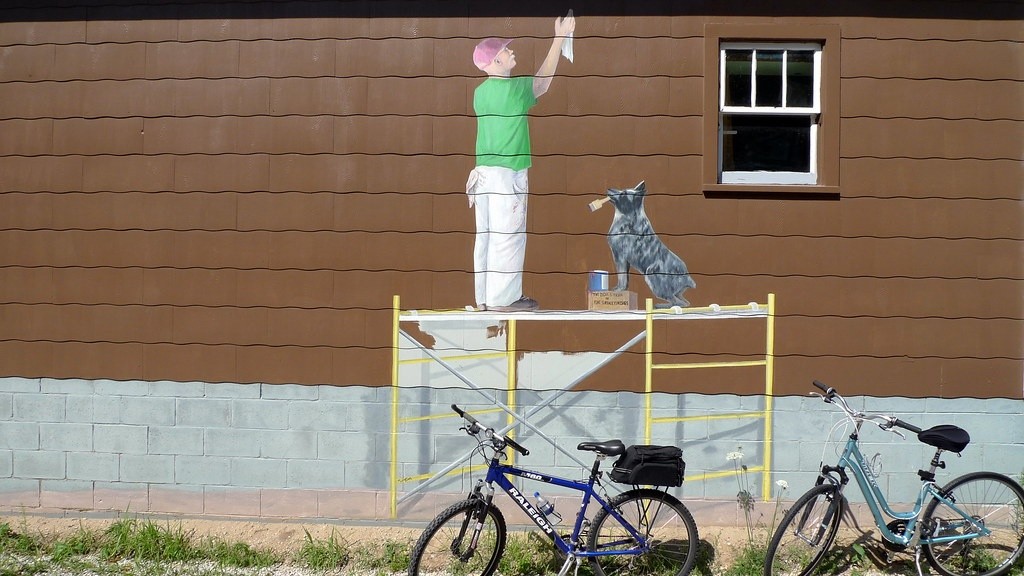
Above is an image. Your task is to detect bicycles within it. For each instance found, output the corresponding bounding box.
[762,379,1024,576]
[406,403,700,576]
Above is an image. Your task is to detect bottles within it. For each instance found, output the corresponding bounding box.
[534,491,562,526]
